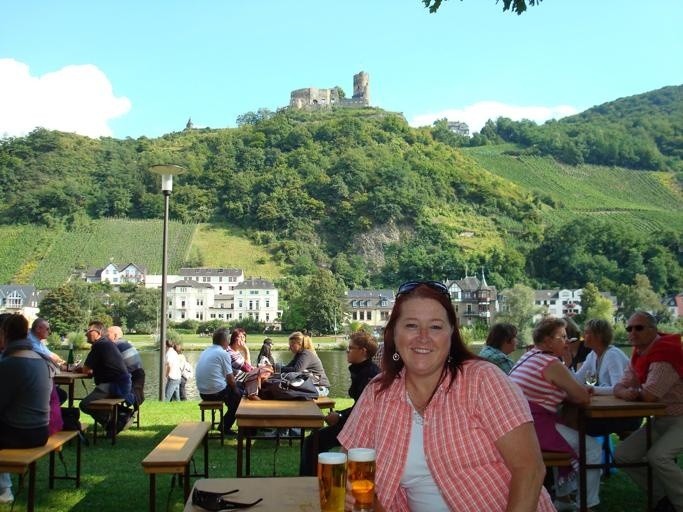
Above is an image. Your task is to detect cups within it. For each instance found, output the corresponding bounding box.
[275,361,281,375]
[318,452,347,512]
[348,448,376,512]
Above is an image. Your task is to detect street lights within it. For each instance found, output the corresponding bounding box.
[148,163,186,401]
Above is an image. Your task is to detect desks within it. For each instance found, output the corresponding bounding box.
[181,476,319,512]
[559,394,668,512]
[235,396,324,478]
[248,373,296,395]
[51,368,93,408]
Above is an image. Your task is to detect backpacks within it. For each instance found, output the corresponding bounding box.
[259,373,319,401]
[182,362,194,380]
[245,366,274,401]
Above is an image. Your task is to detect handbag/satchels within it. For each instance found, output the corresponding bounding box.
[180,358,192,381]
[59,407,90,448]
[507,351,554,376]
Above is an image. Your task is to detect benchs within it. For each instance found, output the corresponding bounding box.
[89,399,140,446]
[141,420,213,511]
[543,451,573,504]
[313,396,336,415]
[198,401,225,449]
[1,422,90,512]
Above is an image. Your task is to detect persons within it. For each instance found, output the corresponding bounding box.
[28,318,70,406]
[258,344,271,368]
[299,331,383,477]
[563,321,643,435]
[195,327,241,437]
[0,365,64,504]
[226,327,256,396]
[335,282,555,512]
[614,311,683,512]
[480,323,518,375]
[173,344,191,401]
[104,326,145,406]
[263,339,272,345]
[507,319,603,510]
[165,340,180,401]
[72,322,132,438]
[558,318,593,374]
[270,332,330,438]
[1,315,50,449]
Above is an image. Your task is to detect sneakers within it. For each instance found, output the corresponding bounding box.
[0,487,15,502]
[280,431,289,436]
[1,472,13,488]
[264,431,278,438]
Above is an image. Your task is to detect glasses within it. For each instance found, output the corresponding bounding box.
[192,487,264,511]
[86,328,101,334]
[625,324,653,332]
[40,327,49,331]
[396,279,447,298]
[346,346,362,353]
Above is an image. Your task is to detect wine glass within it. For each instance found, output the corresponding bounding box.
[585,369,597,386]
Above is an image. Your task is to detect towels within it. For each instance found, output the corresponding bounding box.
[1,339,33,359]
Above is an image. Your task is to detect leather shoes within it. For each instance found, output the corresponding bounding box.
[116,409,137,434]
[655,496,673,511]
[218,425,237,436]
[106,428,120,439]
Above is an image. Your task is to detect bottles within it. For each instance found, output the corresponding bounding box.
[66,343,75,372]
